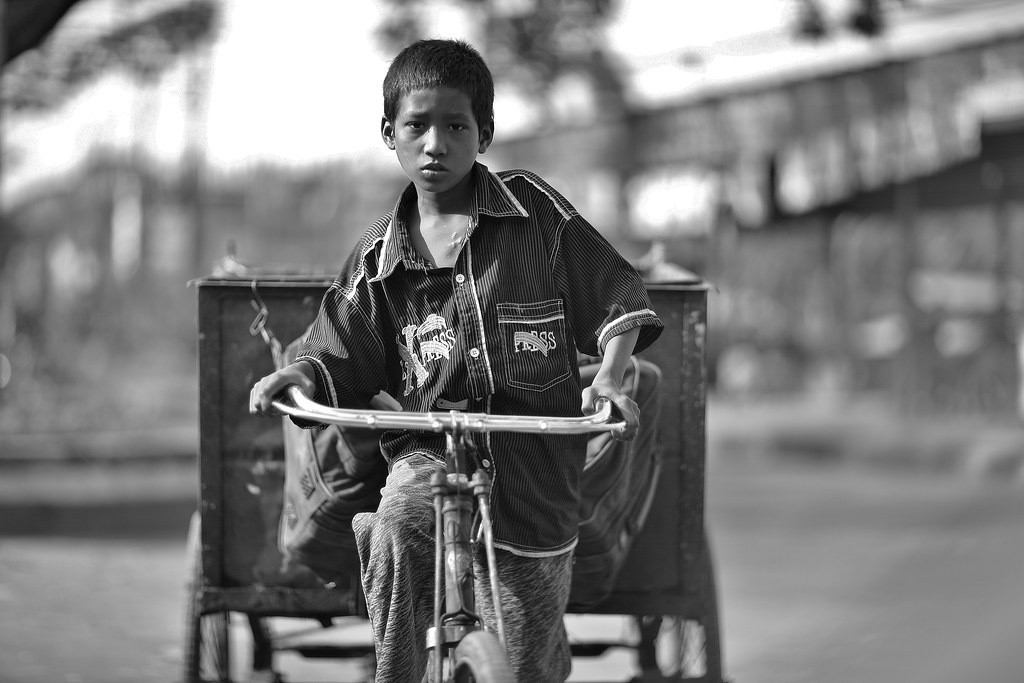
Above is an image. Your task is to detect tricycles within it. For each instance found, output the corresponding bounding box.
[175,260,725,683]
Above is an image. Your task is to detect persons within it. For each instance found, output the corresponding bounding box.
[245,39,662,682]
